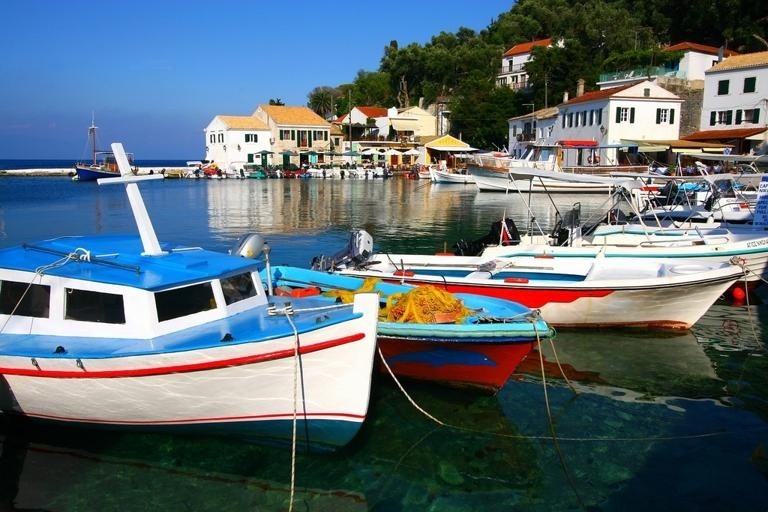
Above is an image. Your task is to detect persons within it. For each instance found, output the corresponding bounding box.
[433,156,437,164]
[444,154,452,167]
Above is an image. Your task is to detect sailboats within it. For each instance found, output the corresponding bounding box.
[73,111,138,182]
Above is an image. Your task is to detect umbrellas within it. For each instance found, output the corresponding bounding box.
[252,148,422,164]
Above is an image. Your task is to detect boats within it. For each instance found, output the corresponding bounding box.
[306,225,751,336]
[256,263,558,393]
[301,159,394,180]
[0,142,380,449]
[417,148,768,288]
